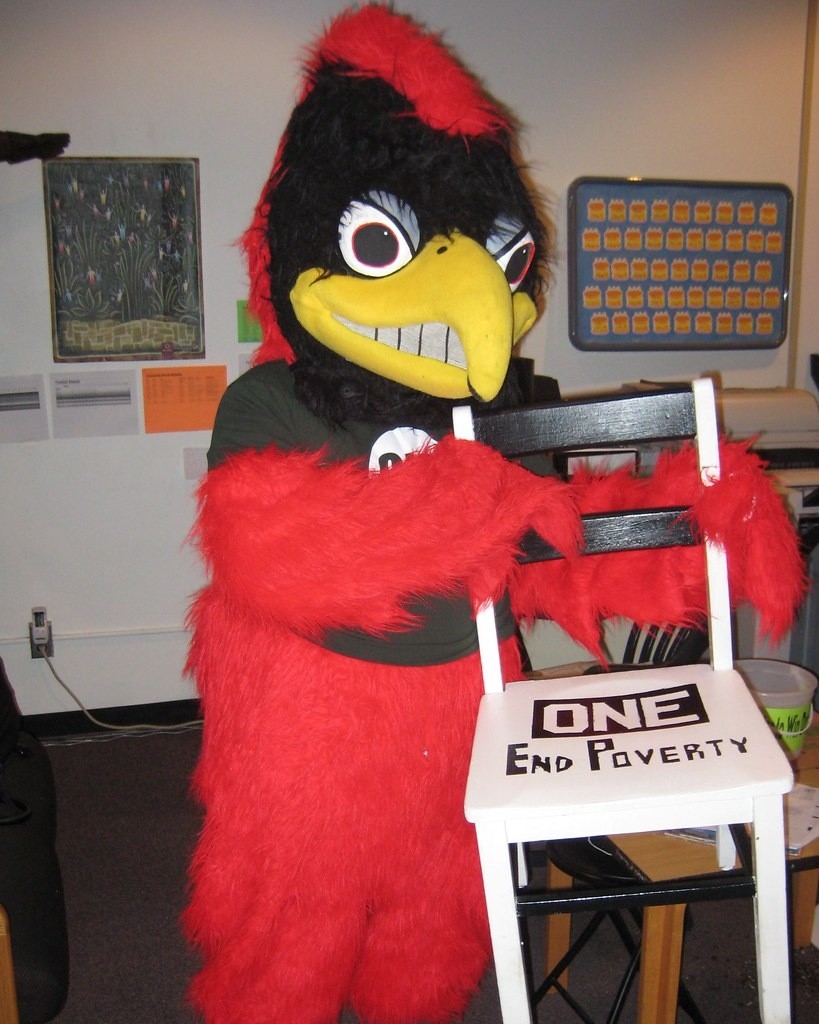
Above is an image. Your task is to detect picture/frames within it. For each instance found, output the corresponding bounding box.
[42,153,206,364]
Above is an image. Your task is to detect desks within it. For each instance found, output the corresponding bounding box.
[538,701,819,1024]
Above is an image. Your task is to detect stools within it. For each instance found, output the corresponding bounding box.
[523,835,707,1024]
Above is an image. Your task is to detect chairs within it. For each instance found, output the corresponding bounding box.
[452,374,793,1024]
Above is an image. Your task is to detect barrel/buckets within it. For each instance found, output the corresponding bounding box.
[733,659,818,762]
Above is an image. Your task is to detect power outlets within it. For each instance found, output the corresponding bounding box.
[29,620,54,659]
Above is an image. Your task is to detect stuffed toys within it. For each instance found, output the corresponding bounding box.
[177,4,805,1024]
